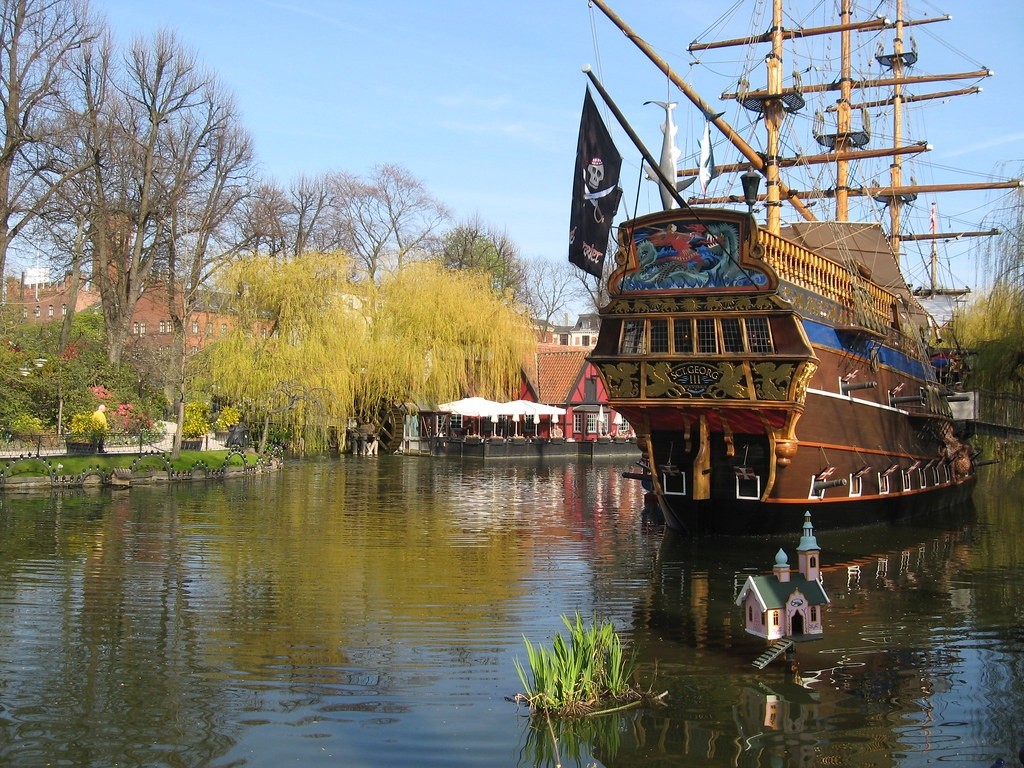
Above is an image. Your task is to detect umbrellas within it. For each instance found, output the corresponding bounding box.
[439,396,568,439]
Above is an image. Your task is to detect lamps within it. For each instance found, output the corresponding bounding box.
[740,166,762,214]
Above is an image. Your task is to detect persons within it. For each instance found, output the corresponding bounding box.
[93,404,108,453]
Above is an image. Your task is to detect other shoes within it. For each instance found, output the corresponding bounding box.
[99,450,107,453]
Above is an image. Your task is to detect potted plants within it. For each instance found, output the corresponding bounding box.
[512,435,525,443]
[532,436,545,443]
[212,406,242,441]
[466,433,480,443]
[597,437,610,444]
[550,436,564,444]
[66,415,111,454]
[489,435,504,442]
[629,437,636,442]
[612,436,627,443]
[174,401,209,450]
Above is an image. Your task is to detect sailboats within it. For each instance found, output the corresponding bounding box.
[564,1,1024,538]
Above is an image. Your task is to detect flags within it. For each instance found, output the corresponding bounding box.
[568,80,624,280]
